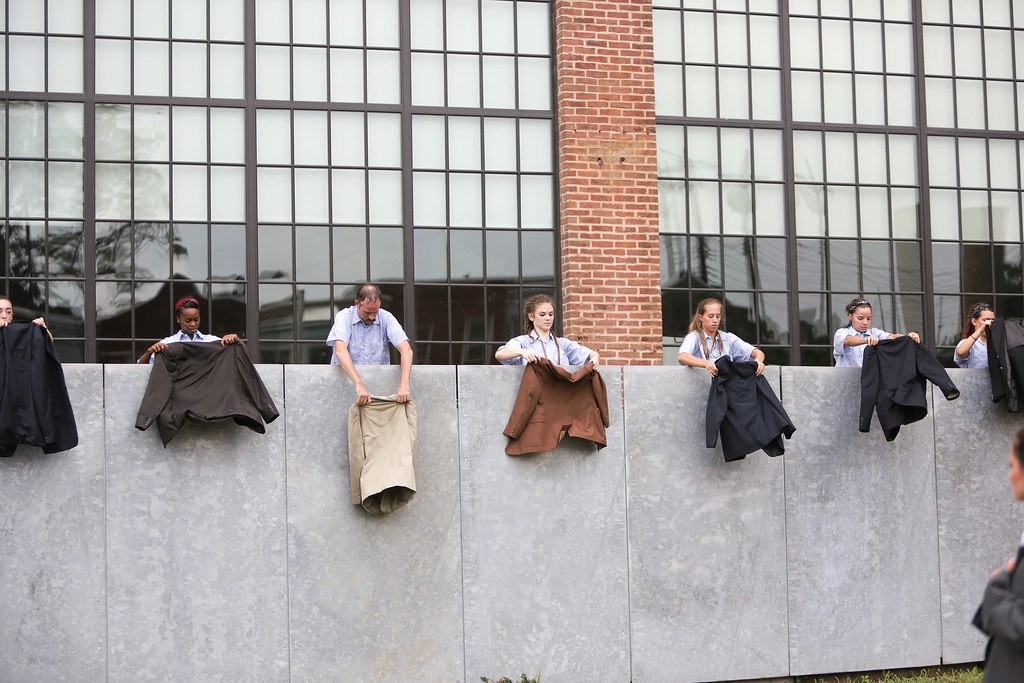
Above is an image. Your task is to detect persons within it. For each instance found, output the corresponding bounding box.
[972,427,1024,683]
[326,284,413,406]
[833,299,920,367]
[0,295,53,343]
[495,295,600,372]
[678,298,765,377]
[137,296,248,364]
[954,303,995,368]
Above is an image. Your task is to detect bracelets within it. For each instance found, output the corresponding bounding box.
[971,335,977,341]
